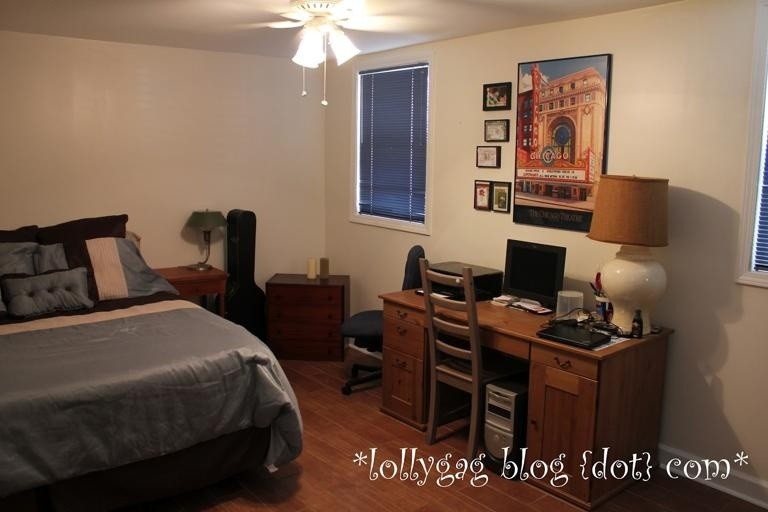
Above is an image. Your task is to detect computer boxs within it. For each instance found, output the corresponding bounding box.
[482,374,527,480]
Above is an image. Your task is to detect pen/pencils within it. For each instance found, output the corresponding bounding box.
[595,272,602,296]
[589,282,598,292]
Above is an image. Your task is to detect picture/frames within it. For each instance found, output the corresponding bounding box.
[513,53,613,231]
[473,82,512,214]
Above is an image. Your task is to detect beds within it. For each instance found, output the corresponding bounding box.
[0,214,304,499]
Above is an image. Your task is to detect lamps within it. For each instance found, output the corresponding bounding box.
[291,0,361,105]
[187,208,229,272]
[585,174,670,335]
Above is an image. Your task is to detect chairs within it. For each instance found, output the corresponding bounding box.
[419,258,525,465]
[339,245,425,396]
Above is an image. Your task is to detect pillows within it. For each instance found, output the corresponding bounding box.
[1,214,179,316]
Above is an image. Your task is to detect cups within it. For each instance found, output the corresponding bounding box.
[594,291,614,323]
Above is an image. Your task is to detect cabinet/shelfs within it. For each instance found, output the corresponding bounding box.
[265,273,350,361]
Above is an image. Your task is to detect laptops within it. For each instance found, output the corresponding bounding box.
[537,320,612,350]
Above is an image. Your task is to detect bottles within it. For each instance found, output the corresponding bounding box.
[319,257,330,280]
[632,309,643,340]
[307,257,317,280]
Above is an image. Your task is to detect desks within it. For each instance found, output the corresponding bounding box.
[378,289,674,511]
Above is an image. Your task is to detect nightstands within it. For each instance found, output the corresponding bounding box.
[150,265,229,318]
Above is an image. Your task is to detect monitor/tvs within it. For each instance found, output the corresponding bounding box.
[502,238,566,313]
